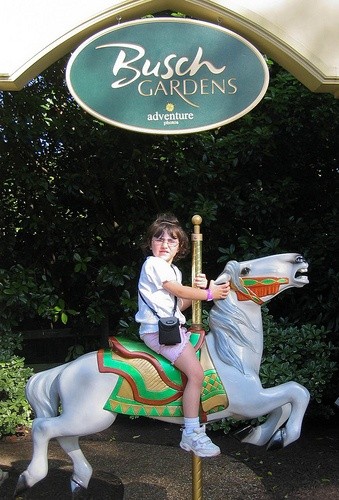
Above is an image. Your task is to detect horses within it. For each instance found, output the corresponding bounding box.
[13,253,310,500]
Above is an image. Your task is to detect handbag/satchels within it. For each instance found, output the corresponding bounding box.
[158,316,182,346]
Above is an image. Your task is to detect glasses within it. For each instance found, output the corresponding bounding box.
[151,238,180,247]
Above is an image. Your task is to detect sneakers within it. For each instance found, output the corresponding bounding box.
[179,425,220,458]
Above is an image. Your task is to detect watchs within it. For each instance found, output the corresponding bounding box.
[205,289,214,301]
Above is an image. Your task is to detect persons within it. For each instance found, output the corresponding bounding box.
[134,211,230,457]
